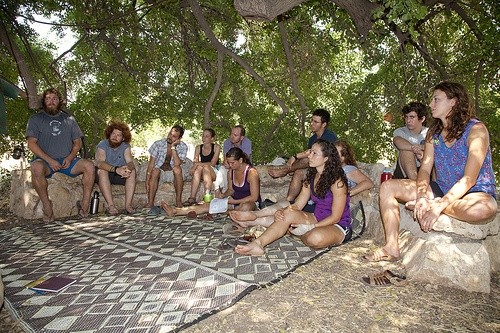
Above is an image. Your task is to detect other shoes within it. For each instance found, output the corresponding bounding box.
[147,206,161,216]
[133,207,152,216]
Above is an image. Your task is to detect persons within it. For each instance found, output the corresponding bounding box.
[163,146,261,216]
[144,125,188,207]
[26,88,95,222]
[183,129,220,205]
[392,103,436,181]
[215,125,252,190]
[229,109,375,255]
[361,82,497,261]
[95,122,135,214]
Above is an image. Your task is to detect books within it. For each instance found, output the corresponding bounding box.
[33,277,77,292]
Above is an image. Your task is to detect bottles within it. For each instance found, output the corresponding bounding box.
[381,167,392,184]
[89,191,99,215]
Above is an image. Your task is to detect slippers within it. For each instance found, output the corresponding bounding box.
[76,200,87,218]
[215,238,250,252]
[360,269,409,287]
[42,200,54,223]
[359,247,401,261]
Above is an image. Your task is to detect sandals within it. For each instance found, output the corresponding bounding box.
[183,197,196,206]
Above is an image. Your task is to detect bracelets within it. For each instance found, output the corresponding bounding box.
[115,166,117,172]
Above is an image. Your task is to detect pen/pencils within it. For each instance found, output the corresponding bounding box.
[218,185,224,197]
